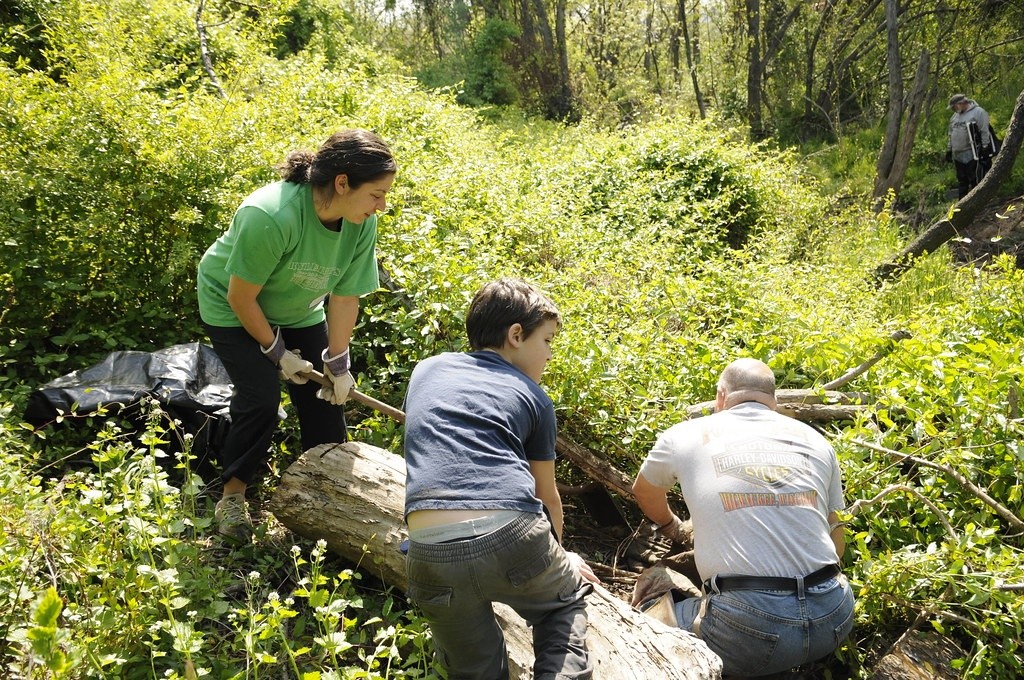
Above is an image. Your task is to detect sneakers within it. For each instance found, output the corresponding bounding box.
[215,493,253,541]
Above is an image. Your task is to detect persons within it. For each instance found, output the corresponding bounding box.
[401,278,600,680]
[632,357,856,677]
[945,94,990,200]
[197,129,397,535]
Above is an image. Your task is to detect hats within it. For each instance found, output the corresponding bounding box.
[945,94,965,110]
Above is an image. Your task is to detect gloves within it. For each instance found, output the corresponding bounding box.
[316,345,357,404]
[260,325,313,385]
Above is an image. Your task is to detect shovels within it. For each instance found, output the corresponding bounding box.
[296,369,636,540]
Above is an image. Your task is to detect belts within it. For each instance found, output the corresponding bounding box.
[704,564,841,592]
